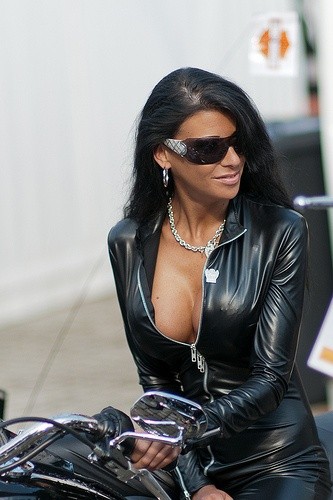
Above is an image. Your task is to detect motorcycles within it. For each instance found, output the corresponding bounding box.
[0,387,333,500]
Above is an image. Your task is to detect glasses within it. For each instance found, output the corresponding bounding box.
[162,128,246,165]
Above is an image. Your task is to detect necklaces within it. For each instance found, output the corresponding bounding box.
[167,197,227,258]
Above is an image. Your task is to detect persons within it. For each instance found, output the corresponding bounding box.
[107,67,332,500]
